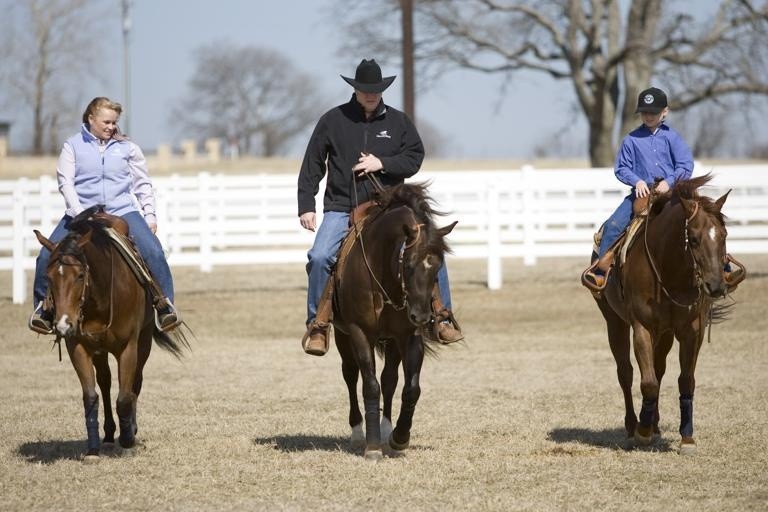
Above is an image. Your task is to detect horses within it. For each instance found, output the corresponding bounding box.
[32,211,195,462]
[590,170,734,452]
[330,179,460,465]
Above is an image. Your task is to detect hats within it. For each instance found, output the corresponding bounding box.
[635,87,667,113]
[341,59,396,93]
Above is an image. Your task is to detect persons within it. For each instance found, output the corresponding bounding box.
[32,97,177,331]
[297,59,465,355]
[585,87,732,286]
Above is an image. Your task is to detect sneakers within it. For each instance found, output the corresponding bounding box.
[158,313,176,328]
[586,271,605,300]
[438,321,464,342]
[32,318,51,331]
[724,269,741,293]
[305,333,326,354]
